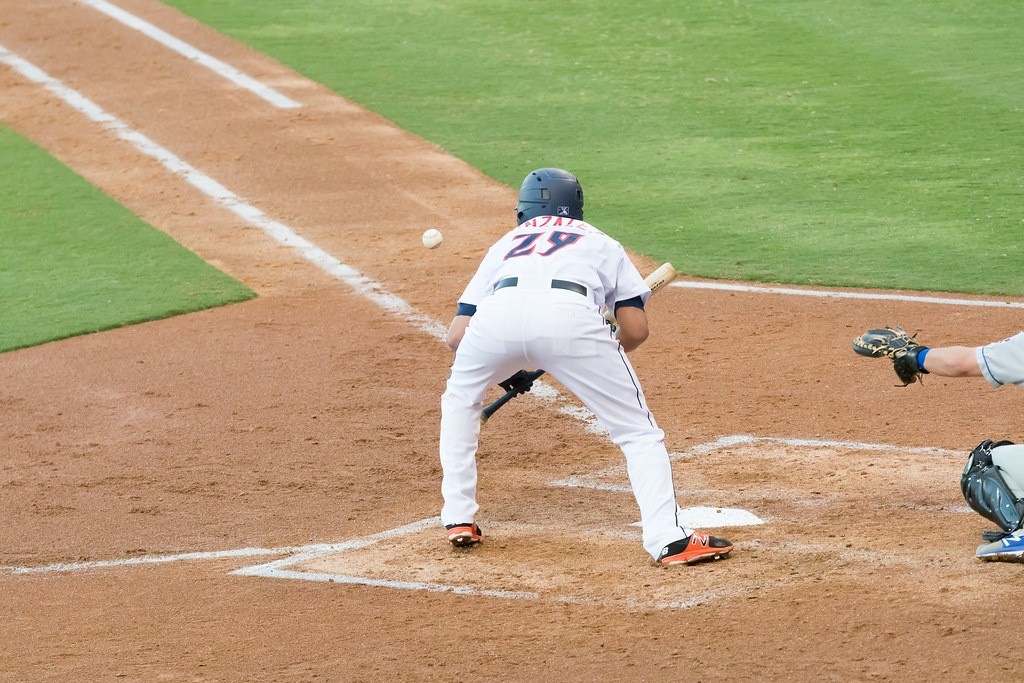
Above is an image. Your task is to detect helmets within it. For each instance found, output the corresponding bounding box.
[516,168,583,225]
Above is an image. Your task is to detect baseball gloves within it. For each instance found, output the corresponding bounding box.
[855,328,920,389]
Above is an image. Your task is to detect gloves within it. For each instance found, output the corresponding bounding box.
[499,370,532,396]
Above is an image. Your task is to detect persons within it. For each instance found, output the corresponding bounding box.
[437,168,735,567]
[849,325,1024,558]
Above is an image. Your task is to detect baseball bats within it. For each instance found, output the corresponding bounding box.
[480,262,679,424]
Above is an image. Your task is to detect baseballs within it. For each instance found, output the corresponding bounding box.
[421,226,444,252]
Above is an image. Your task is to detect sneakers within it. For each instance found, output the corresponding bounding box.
[976,530,1024,558]
[659,530,734,566]
[445,522,484,547]
